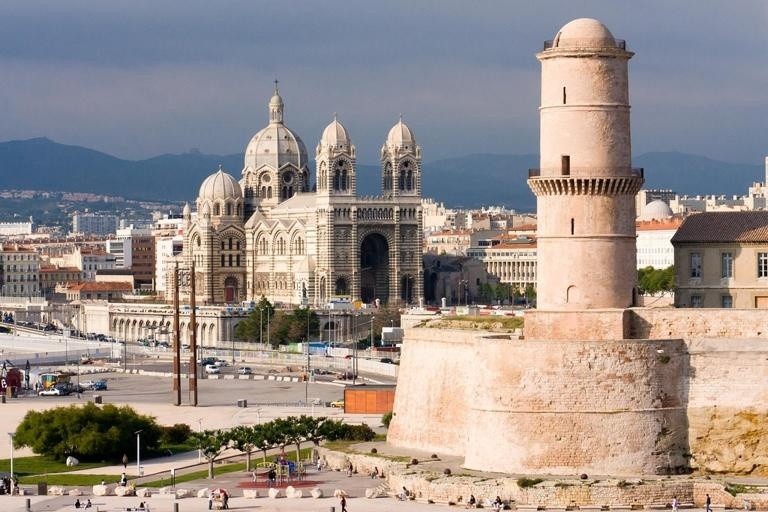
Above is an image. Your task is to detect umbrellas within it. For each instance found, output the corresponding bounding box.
[210,487,227,494]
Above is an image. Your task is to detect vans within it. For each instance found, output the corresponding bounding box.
[205,364,220,374]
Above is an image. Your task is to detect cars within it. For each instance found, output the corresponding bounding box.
[237,366,252,375]
[37,387,69,396]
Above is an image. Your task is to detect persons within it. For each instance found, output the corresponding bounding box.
[346,461,354,477]
[704,493,712,511]
[401,486,408,500]
[119,473,127,486]
[138,502,145,511]
[316,456,322,472]
[101,478,107,485]
[491,496,501,511]
[671,495,678,512]
[122,453,129,468]
[266,466,277,487]
[144,501,150,512]
[253,467,257,482]
[465,495,476,509]
[371,466,378,479]
[208,491,229,511]
[85,499,91,508]
[75,498,80,507]
[340,496,347,512]
[0,473,20,496]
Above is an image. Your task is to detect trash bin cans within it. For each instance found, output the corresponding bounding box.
[330,507,335,512]
[743,499,752,510]
[27,499,31,512]
[174,503,178,512]
[94,395,103,403]
[2,395,6,403]
[238,399,247,407]
[140,466,144,476]
[326,402,331,406]
